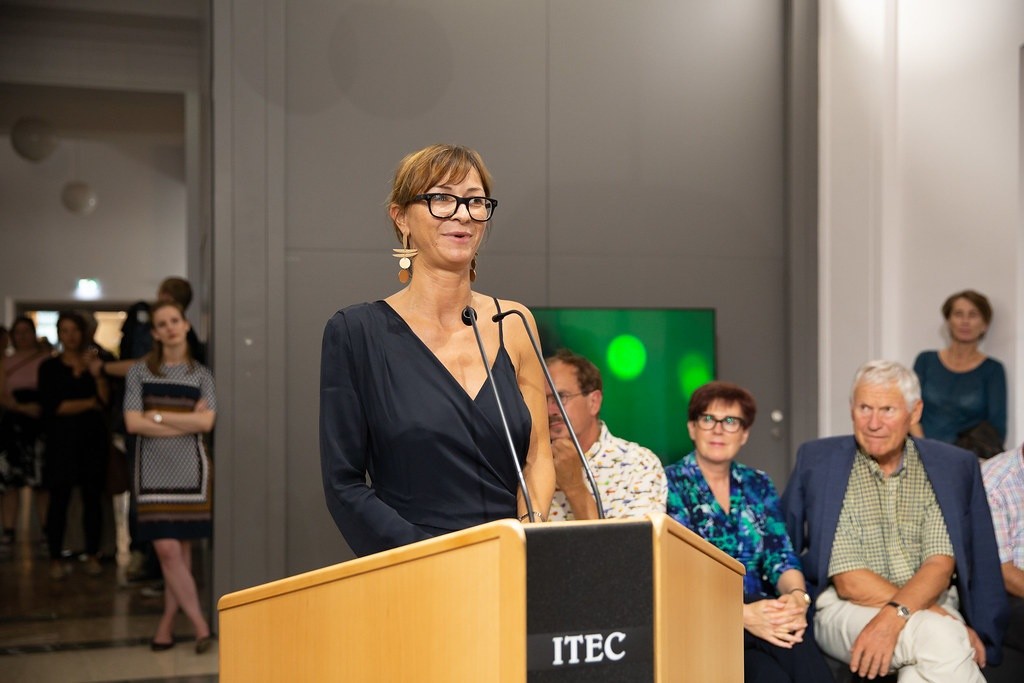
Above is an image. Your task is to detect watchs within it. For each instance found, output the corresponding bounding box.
[886,602,912,619]
[154,410,162,424]
[788,588,812,610]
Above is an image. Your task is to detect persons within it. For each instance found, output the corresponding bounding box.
[662,381,840,683]
[546,351,669,524]
[122,301,219,656]
[33,311,128,587]
[979,442,1024,682]
[318,142,555,558]
[777,359,1011,683]
[78,308,125,441]
[1,314,49,548]
[913,290,1007,461]
[88,274,209,601]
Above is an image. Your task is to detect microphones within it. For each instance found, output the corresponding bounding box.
[491,309,605,520]
[460,306,534,524]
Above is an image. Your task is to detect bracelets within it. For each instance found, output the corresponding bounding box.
[100,363,106,377]
[517,511,547,524]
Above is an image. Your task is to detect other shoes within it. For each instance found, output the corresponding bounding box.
[80,559,104,575]
[49,565,65,578]
[152,632,175,651]
[196,633,215,654]
[141,581,165,596]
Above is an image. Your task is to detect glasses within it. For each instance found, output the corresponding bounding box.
[697,413,744,432]
[414,193,498,222]
[546,392,582,406]
